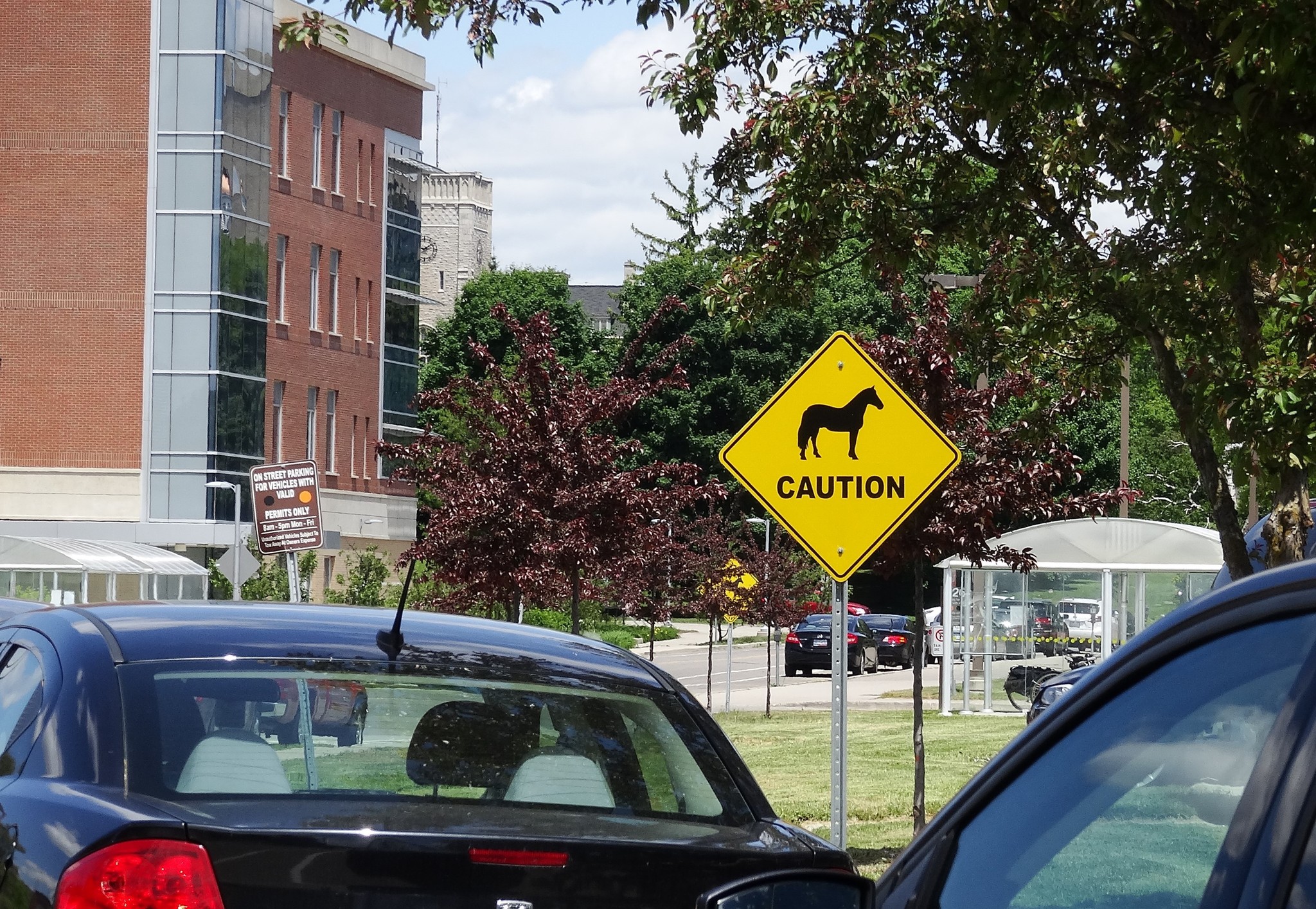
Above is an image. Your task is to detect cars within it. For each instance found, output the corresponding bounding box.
[0,596,874,909]
[695,553,1315,908]
[762,589,1134,676]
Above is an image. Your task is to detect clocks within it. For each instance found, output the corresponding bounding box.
[420,236,437,263]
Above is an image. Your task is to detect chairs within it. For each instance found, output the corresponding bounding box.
[174,724,297,798]
[501,748,617,808]
[404,700,516,802]
